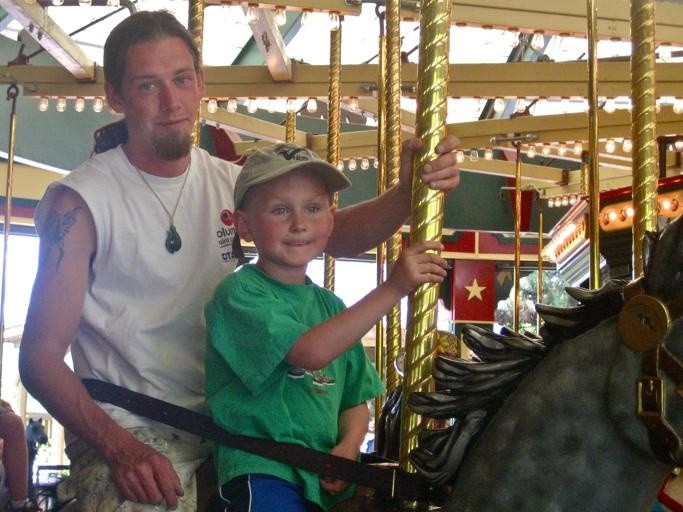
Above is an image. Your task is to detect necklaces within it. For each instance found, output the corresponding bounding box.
[134,161,192,253]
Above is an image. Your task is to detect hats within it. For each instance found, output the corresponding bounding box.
[233,142,351,208]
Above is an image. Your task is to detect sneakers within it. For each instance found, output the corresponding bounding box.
[5,501,43,511]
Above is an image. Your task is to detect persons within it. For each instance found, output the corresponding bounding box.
[203,141,447,512]
[18,9,460,512]
[0,397,39,512]
